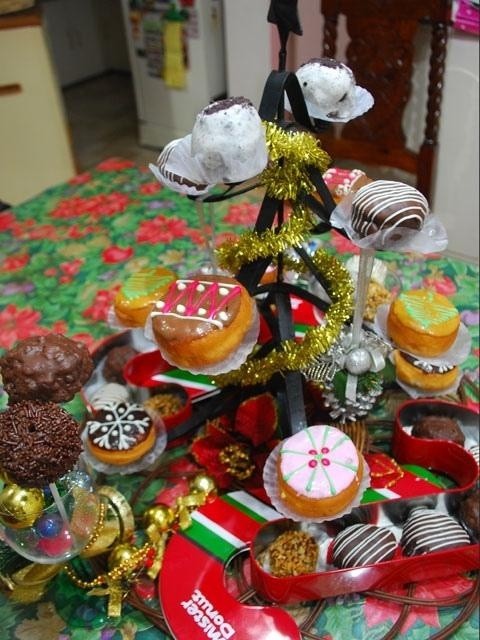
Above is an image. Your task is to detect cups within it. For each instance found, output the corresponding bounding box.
[0,453,106,627]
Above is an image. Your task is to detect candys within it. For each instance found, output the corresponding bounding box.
[0,480,97,556]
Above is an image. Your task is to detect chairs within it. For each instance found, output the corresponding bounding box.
[310,2,447,208]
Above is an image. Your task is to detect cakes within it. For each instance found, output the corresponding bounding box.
[192,99,265,183]
[0,404,81,554]
[153,276,253,367]
[461,489,479,534]
[86,405,155,466]
[387,289,460,358]
[277,425,364,517]
[412,415,463,450]
[114,267,177,327]
[331,524,397,568]
[351,180,429,250]
[143,395,184,420]
[103,347,141,383]
[269,530,318,580]
[401,512,472,556]
[394,349,459,392]
[295,60,357,116]
[92,383,129,412]
[0,337,92,406]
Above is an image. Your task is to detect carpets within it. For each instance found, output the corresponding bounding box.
[1,154,477,640]
